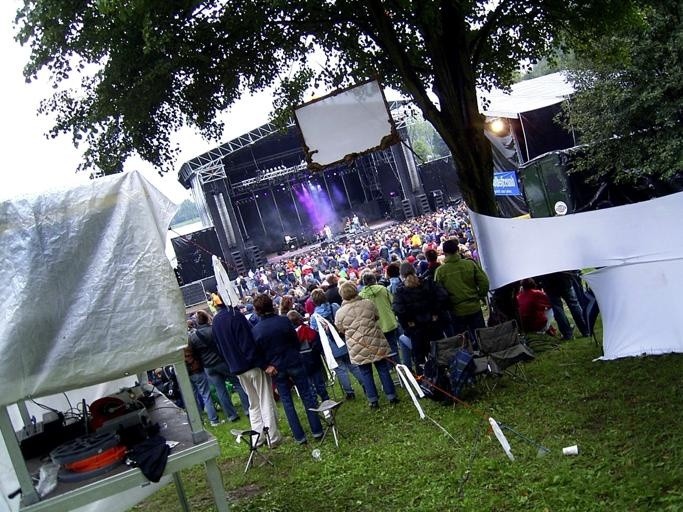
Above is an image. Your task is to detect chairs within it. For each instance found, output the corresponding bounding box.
[423,331,475,400]
[471,318,531,392]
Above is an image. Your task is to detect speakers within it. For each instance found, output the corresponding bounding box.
[416,194,431,214]
[248,245,264,268]
[430,189,446,208]
[321,242,328,247]
[401,199,414,218]
[339,236,347,242]
[231,250,246,275]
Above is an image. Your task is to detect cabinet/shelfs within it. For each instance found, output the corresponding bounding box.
[0,167,231,512]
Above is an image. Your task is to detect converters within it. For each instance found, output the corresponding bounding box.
[42,411,63,431]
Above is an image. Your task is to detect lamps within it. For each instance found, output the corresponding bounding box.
[257,164,286,174]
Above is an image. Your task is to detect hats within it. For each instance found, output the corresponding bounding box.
[196,310,209,326]
[400,262,416,279]
[442,239,459,255]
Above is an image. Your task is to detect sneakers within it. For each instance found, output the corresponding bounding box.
[369,399,380,410]
[298,440,308,445]
[389,397,402,404]
[267,435,292,449]
[346,393,356,401]
[314,432,325,441]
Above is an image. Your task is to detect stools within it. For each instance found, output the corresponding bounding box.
[305,399,343,448]
[227,427,276,475]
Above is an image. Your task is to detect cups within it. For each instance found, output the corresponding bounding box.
[563,444,578,456]
[312,448,322,462]
